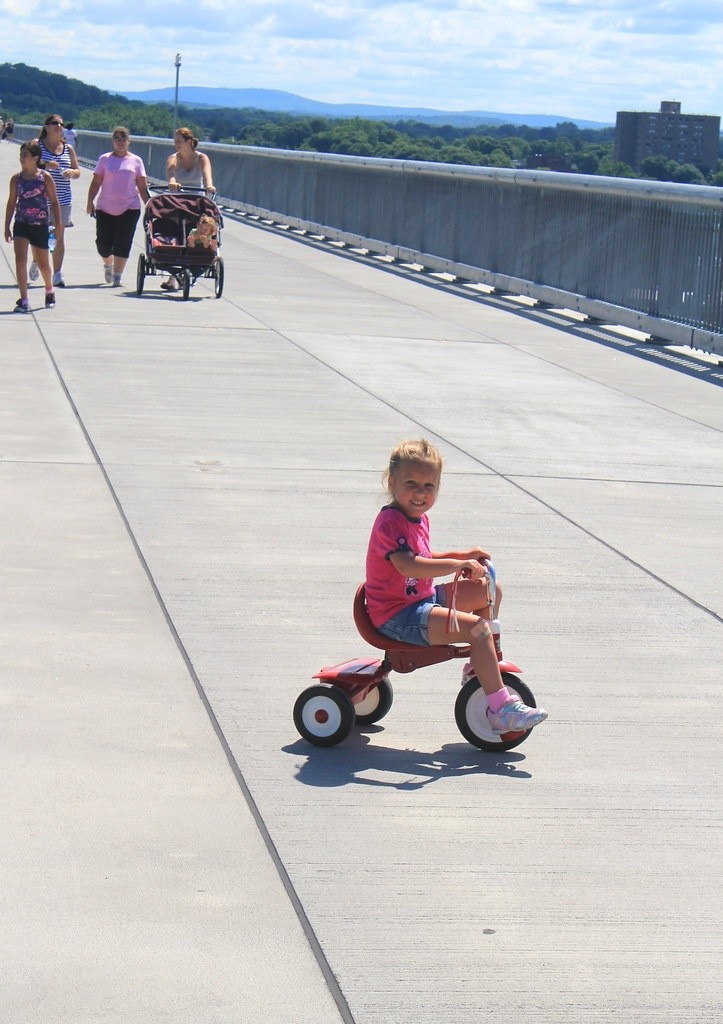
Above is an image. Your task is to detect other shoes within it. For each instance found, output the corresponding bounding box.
[113,279,122,287]
[161,283,176,291]
[103,264,113,282]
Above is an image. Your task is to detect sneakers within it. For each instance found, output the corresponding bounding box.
[487,694,549,734]
[52,272,64,286]
[13,299,29,312]
[460,662,478,685]
[44,292,56,307]
[29,263,40,281]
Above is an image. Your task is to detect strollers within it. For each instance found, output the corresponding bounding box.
[138,189,224,301]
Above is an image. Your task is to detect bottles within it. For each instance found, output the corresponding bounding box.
[59,166,69,180]
[48,225,58,252]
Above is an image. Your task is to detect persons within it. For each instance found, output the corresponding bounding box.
[365,436,548,734]
[29,114,80,286]
[160,127,216,289]
[0,116,15,140]
[86,126,151,288]
[63,123,79,149]
[4,141,63,312]
[187,213,218,252]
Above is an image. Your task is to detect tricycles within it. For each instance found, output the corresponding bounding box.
[293,581,537,753]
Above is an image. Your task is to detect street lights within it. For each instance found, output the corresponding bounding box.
[174,54,182,139]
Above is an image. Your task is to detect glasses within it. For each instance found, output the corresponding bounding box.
[113,136,126,142]
[48,121,64,127]
